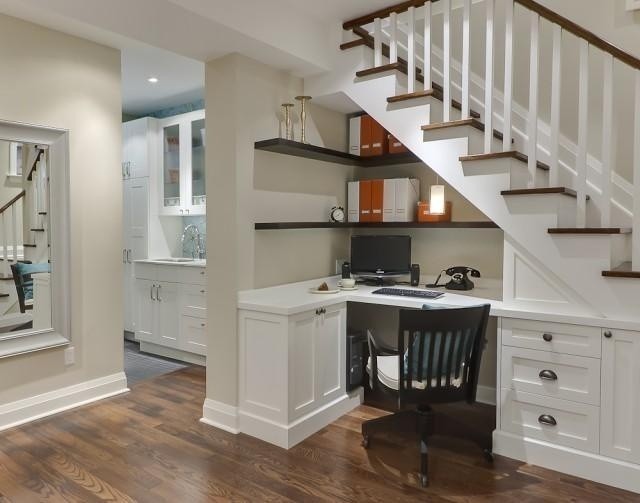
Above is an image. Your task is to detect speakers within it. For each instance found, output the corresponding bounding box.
[342,264,350,279]
[411,264,419,286]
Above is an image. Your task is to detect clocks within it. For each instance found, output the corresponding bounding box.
[330,205,347,222]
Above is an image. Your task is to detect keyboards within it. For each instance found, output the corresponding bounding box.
[372,288,445,299]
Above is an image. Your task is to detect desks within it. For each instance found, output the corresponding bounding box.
[235,277,499,448]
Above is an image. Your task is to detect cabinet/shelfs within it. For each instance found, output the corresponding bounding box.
[124,177,182,333]
[181,266,209,357]
[493,315,601,452]
[365,153,500,228]
[27,271,54,330]
[122,116,162,177]
[155,108,207,215]
[601,327,640,466]
[253,136,365,230]
[135,261,182,350]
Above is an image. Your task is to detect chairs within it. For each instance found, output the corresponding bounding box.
[8,262,33,313]
[359,303,495,489]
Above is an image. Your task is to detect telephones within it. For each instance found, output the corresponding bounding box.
[445,266,480,290]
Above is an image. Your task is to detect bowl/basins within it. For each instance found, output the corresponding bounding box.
[164,197,181,205]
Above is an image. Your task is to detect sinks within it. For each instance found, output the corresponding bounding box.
[148,258,193,263]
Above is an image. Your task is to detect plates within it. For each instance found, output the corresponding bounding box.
[336,286,358,290]
[308,285,340,293]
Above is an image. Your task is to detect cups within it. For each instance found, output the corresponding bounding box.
[197,233,206,258]
[337,278,356,287]
[169,168,179,183]
[200,127,205,144]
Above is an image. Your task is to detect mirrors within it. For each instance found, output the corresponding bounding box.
[1,119,72,358]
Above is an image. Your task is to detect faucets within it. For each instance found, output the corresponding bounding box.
[181,223,206,260]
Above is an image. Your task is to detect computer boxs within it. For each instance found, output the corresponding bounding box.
[346,332,364,390]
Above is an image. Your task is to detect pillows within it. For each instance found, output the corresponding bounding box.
[15,262,50,303]
[398,300,489,380]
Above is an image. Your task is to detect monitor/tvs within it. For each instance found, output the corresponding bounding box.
[351,234,411,286]
